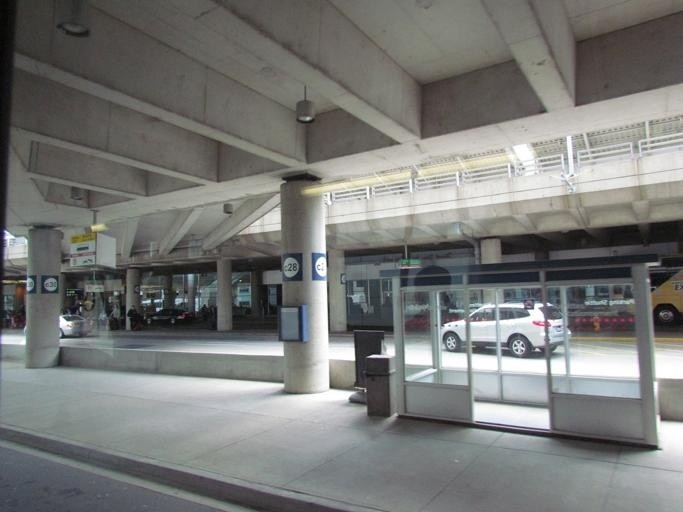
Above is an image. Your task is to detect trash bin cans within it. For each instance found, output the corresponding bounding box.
[366,355,397,417]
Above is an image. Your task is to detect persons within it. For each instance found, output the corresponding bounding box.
[126,305,139,331]
[97,308,107,331]
[108,303,122,331]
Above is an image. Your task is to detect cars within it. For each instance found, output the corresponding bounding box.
[145,308,187,325]
[24,315,88,338]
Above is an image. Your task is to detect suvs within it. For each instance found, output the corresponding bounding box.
[440,302,571,357]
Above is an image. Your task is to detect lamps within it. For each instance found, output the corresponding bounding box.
[70,187,82,201]
[296,86,316,124]
[57,0,91,37]
[224,201,232,215]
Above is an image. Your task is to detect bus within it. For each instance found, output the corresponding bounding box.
[624,267,682,326]
